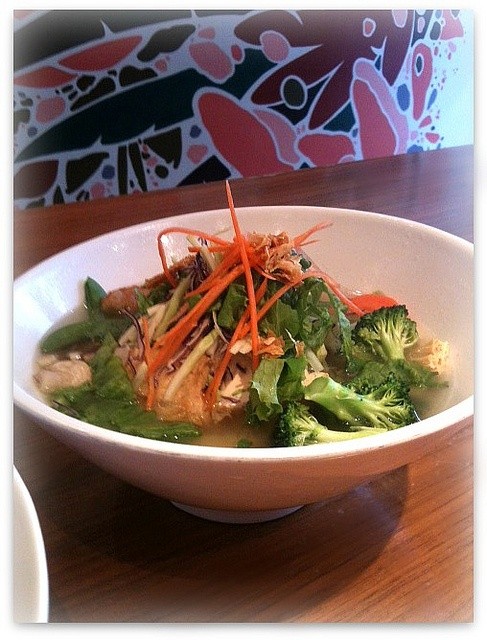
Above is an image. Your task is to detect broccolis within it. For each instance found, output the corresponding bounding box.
[302,374,416,432]
[354,303,420,364]
[275,403,382,448]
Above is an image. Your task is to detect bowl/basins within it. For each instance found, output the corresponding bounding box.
[13,205,475,524]
